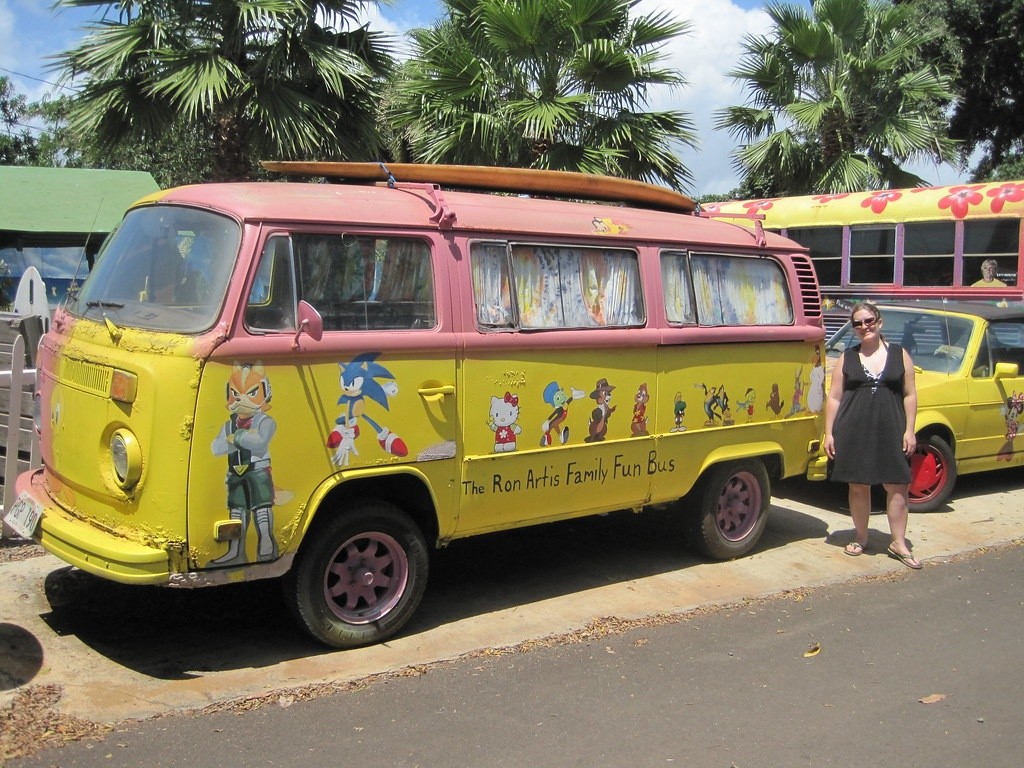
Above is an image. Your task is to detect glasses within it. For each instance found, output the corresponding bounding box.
[852,318,878,328]
[983,266,995,271]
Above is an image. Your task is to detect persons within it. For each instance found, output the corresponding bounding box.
[821,304,922,569]
[970,259,1010,288]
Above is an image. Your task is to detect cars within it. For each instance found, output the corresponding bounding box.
[825,293,1024,512]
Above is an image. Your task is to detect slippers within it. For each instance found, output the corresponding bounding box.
[843,541,868,556]
[887,544,923,569]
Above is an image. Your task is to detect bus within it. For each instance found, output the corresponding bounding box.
[691,179,1024,357]
[6,173,829,649]
